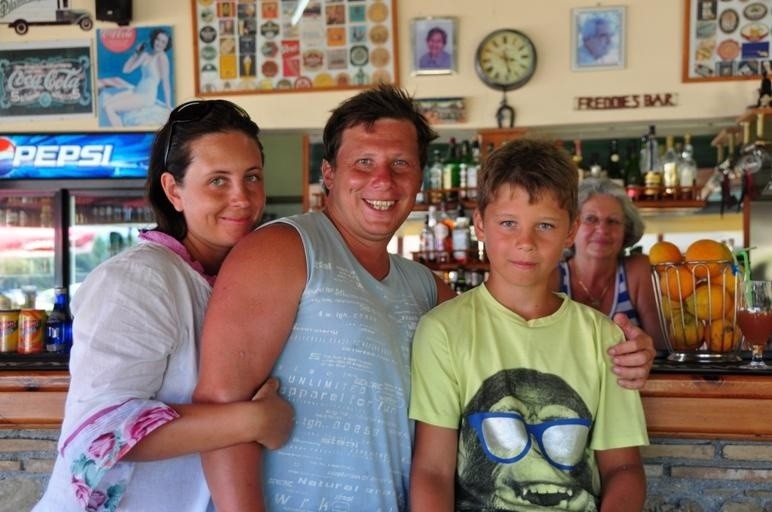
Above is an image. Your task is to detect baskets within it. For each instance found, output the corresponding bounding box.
[649,259,745,353]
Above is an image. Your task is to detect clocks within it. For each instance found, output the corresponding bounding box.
[474,28,537,92]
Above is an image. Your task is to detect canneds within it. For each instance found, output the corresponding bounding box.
[17,309,48,355]
[643,170,661,198]
[0,308,20,353]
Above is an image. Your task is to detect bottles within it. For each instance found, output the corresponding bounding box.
[426,135,510,201]
[550,124,699,200]
[434,265,484,296]
[75,195,159,224]
[414,201,489,263]
[0,197,55,227]
[46,286,74,360]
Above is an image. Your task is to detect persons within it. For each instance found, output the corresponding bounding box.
[405,138,652,512]
[102,27,172,127]
[191,84,657,512]
[31,99,296,512]
[418,27,451,68]
[547,175,665,353]
[578,17,614,64]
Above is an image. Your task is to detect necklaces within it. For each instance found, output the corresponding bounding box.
[578,279,611,307]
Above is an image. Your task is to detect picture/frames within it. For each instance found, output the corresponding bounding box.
[189,0,401,97]
[571,5,627,72]
[681,1,771,86]
[409,15,459,76]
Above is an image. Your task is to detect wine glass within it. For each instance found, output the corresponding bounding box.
[735,278,771,371]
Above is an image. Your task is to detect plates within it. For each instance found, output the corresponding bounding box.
[198,2,392,94]
[717,1,769,59]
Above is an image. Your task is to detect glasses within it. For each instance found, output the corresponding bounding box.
[464,412,594,473]
[163,98,251,170]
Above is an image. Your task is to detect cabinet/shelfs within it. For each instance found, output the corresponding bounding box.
[298,118,772,356]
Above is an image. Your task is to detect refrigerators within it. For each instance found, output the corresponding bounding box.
[0,129,309,317]
